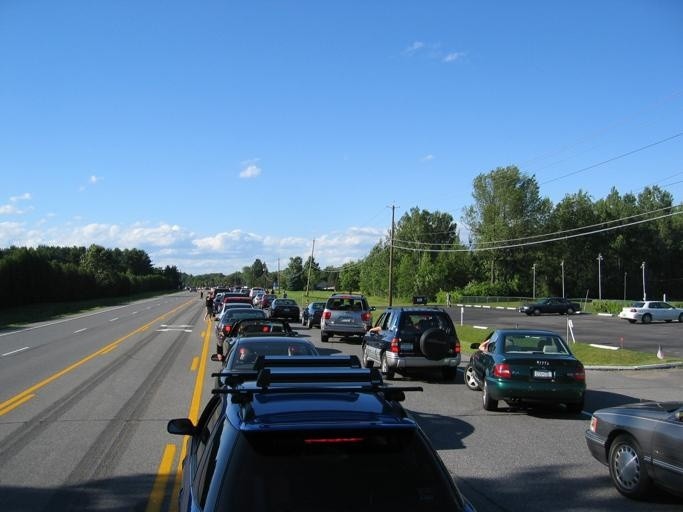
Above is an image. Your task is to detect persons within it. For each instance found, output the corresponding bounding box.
[368,325,382,333]
[478,338,490,352]
[203,291,214,322]
[282,291,286,298]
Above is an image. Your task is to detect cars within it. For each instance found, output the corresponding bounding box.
[464,327,587,415]
[620,301,683,324]
[516,296,581,316]
[585,400,683,499]
